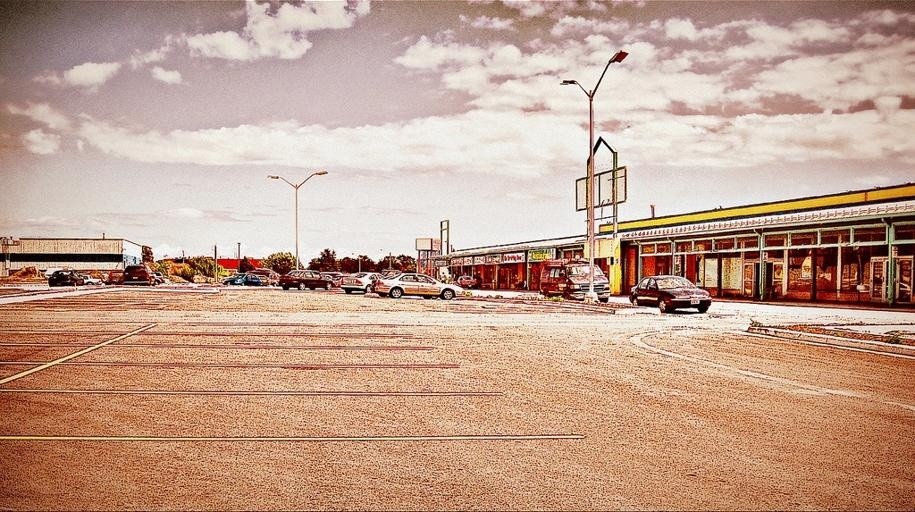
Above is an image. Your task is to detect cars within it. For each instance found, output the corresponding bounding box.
[784,271,911,301]
[219,265,483,302]
[627,273,714,315]
[46,261,168,288]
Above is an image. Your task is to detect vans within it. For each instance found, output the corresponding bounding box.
[538,258,613,306]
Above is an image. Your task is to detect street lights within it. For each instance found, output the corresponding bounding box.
[350,251,362,272]
[266,170,329,272]
[558,47,630,306]
[379,248,393,270]
